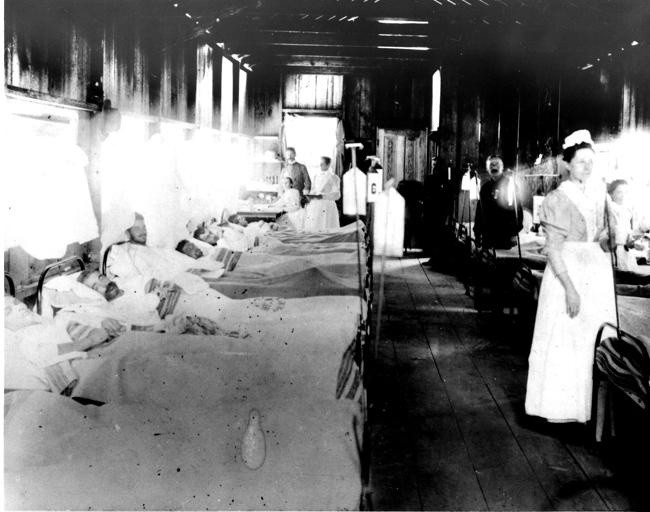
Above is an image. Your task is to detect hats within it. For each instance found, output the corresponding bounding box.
[561,129,594,150]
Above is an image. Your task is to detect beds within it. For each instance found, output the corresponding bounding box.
[453,219,650,439]
[5,209,375,510]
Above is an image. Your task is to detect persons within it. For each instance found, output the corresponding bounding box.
[472,151,524,254]
[609,179,650,273]
[521,129,624,444]
[4,145,359,511]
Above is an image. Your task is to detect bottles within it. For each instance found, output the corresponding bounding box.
[240,408,267,470]
